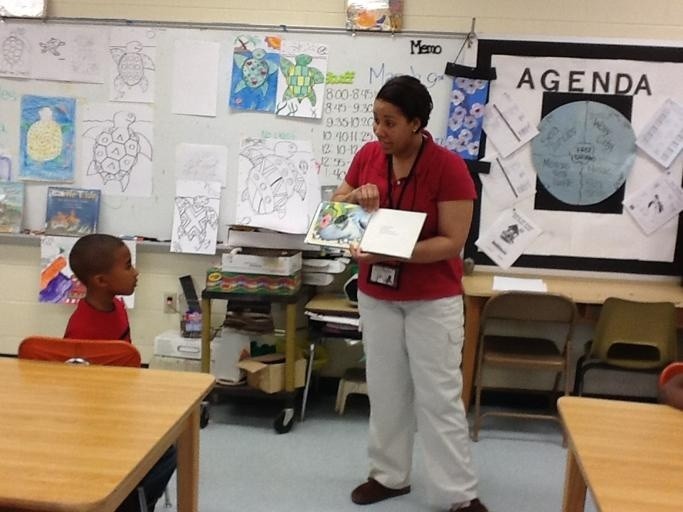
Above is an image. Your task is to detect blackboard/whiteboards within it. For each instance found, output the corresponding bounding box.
[1,16,479,258]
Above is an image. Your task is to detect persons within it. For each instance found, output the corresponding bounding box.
[62,231,179,512]
[329,74,495,512]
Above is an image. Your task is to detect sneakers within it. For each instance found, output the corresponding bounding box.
[451,498,489,511]
[352,477,411,505]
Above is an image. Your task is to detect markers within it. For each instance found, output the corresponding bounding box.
[117,235,143,241]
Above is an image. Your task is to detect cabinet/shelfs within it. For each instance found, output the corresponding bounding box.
[200,284,302,434]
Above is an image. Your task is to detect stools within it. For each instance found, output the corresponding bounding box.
[333,368,378,416]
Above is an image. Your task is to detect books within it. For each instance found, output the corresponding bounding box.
[303,200,428,262]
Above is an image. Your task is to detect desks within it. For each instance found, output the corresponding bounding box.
[1,357,216,511]
[555,397,683,511]
[300,293,360,419]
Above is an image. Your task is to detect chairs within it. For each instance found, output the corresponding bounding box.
[463,271,682,442]
[18,335,180,512]
